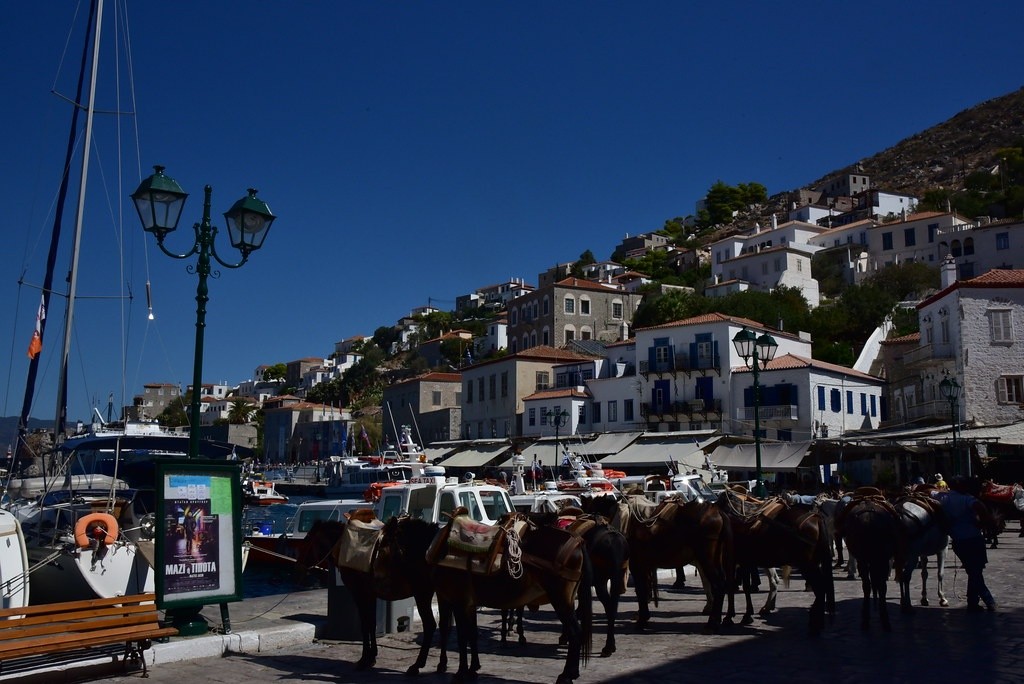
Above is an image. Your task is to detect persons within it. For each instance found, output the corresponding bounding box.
[915,472,950,491]
[183,511,197,554]
[930,475,998,612]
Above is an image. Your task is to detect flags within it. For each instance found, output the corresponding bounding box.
[35,294,46,335]
[25,332,42,359]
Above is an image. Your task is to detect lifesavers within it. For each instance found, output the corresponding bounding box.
[604,469,627,478]
[73,513,120,547]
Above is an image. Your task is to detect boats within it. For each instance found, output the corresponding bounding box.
[0,442,704,624]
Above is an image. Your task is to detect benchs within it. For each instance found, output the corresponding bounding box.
[0,595,180,684]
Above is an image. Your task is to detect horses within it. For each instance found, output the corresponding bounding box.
[289,473,1024,684]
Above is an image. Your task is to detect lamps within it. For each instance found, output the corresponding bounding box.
[937,306,947,316]
[925,372,935,380]
[922,315,931,323]
[940,367,951,375]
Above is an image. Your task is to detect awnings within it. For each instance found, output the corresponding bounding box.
[405,445,459,462]
[594,434,723,466]
[582,432,642,454]
[498,440,593,471]
[703,440,811,473]
[436,445,513,467]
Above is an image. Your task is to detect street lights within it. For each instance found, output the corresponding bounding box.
[731,323,779,497]
[940,374,961,446]
[127,158,279,458]
[545,407,571,471]
